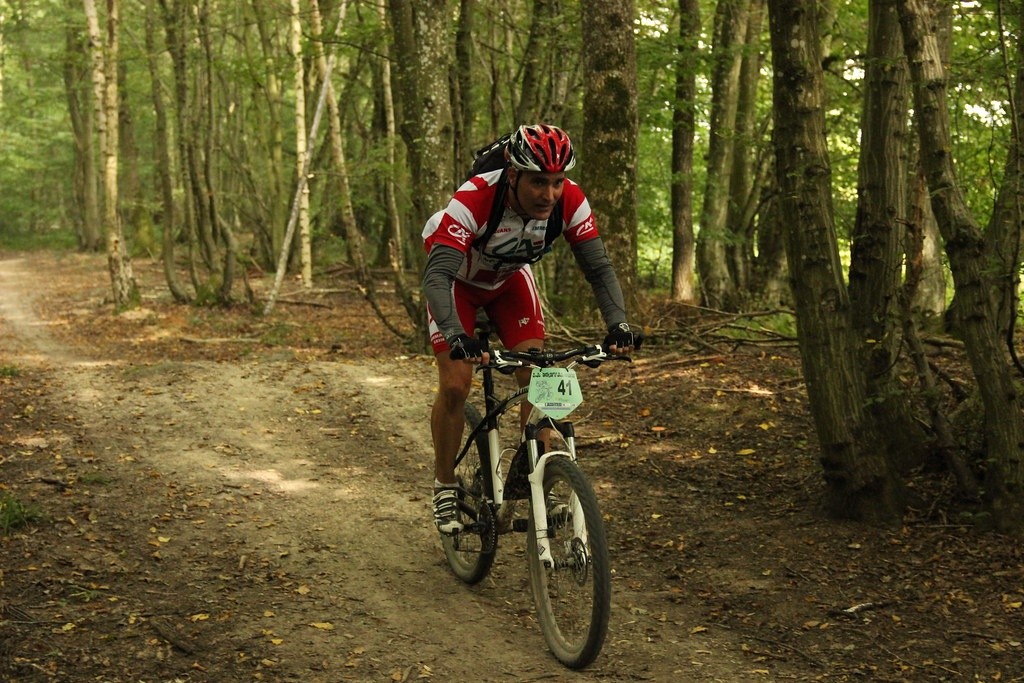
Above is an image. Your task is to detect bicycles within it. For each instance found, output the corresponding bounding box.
[438,328,632,671]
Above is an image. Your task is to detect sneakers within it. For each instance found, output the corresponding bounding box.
[432,484,464,534]
[545,491,572,520]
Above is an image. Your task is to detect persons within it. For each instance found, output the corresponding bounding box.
[421,125,636,537]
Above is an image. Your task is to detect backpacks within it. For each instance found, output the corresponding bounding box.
[463,132,564,251]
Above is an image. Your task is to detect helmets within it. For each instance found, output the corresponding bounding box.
[505,125,575,171]
